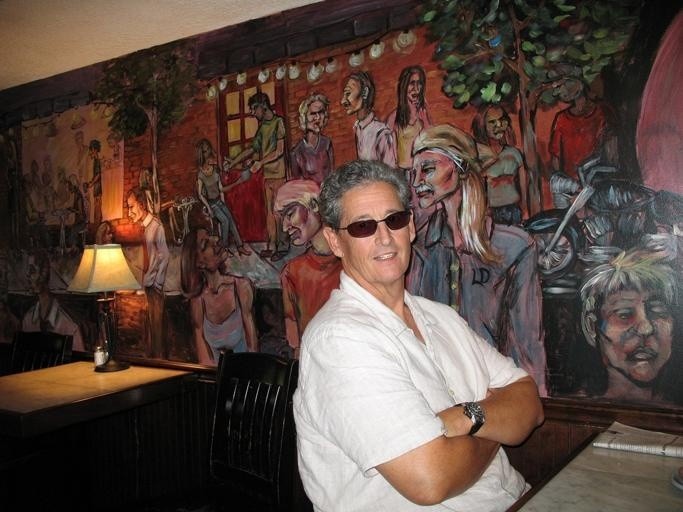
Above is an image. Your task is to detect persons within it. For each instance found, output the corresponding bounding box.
[290,156,546,512]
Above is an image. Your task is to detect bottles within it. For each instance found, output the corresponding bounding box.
[94,340,109,366]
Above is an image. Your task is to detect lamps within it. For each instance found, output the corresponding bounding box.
[67,244,141,372]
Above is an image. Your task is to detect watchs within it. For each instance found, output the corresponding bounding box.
[453,401,486,437]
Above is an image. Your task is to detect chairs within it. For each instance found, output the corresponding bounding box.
[167,349,298,512]
[78,198,91,248]
[6,331,73,375]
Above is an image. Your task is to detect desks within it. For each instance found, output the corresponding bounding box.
[1,361,201,512]
[51,210,73,257]
[504,426,682,512]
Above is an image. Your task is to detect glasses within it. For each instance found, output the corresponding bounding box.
[336,211,412,238]
